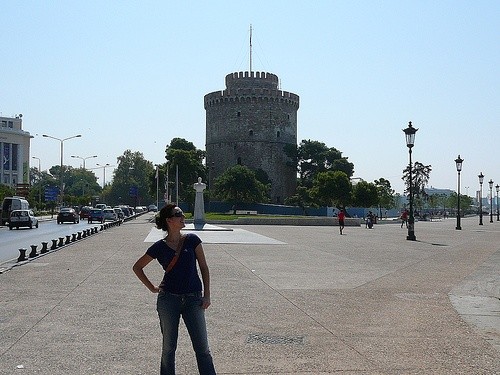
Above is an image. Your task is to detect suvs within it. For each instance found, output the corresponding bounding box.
[80,206,92,220]
[8,210,39,229]
[95,204,107,210]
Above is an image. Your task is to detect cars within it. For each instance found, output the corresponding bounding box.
[107,205,157,220]
[87,209,105,224]
[57,208,79,224]
[103,209,118,221]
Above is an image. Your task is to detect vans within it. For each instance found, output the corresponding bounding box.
[0,196,30,226]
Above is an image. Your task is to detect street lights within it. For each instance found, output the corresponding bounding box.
[494,183,500,221]
[96,163,110,187]
[43,134,82,210]
[401,121,420,241]
[70,155,97,169]
[478,172,485,226]
[32,157,40,173]
[488,179,494,223]
[454,154,464,230]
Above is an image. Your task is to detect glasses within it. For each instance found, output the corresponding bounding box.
[167,210,183,217]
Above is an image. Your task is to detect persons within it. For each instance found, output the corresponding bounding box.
[366,211,374,228]
[407,210,410,221]
[446,210,449,218]
[194,177,206,192]
[443,209,446,219]
[401,209,407,228]
[338,209,345,235]
[132,202,217,375]
